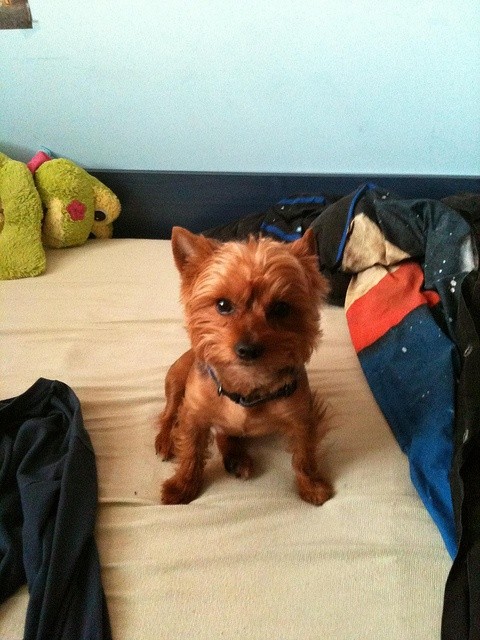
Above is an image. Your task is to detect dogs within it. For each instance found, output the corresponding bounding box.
[155,226,341,507]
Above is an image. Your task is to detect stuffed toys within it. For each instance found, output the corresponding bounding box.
[0,150,122,280]
